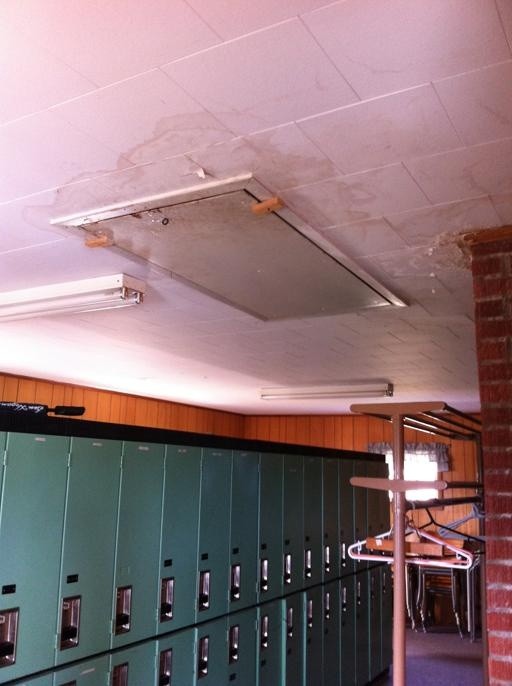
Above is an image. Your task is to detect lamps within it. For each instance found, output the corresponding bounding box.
[1,274,146,325]
[261,381,393,398]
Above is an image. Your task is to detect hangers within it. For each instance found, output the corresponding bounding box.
[348,499,484,570]
